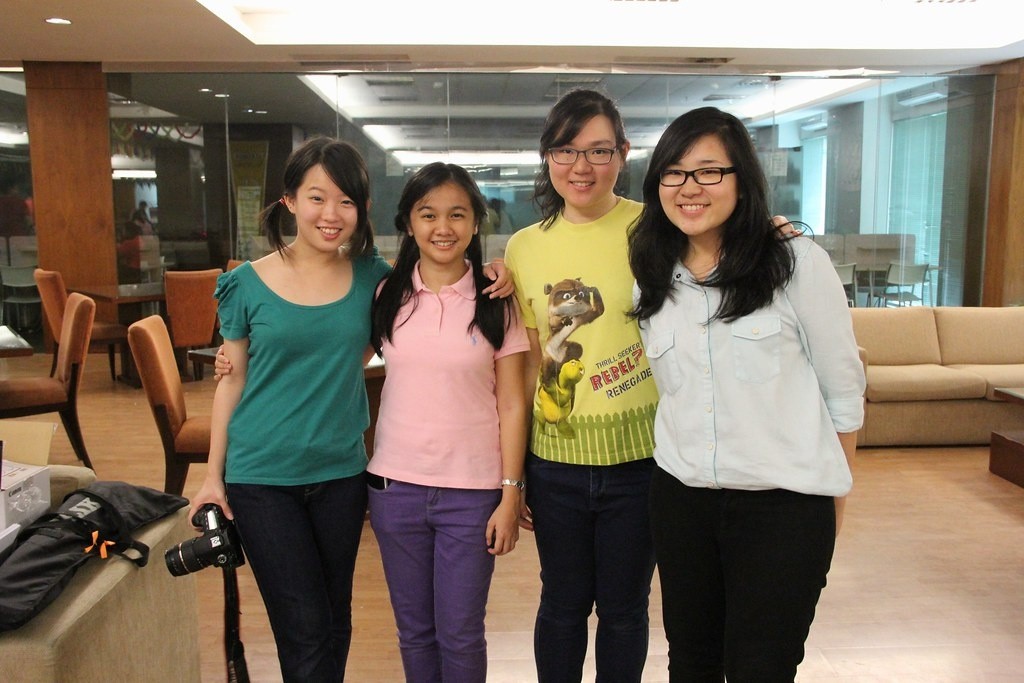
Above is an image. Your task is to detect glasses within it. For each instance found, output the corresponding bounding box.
[546,144,620,164]
[659,167,735,187]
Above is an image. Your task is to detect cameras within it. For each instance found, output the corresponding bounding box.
[165,503,246,577]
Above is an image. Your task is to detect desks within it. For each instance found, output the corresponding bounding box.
[65,281,166,388]
[187,345,386,377]
[0,325,33,358]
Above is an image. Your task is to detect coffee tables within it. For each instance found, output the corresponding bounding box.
[989,388,1024,489]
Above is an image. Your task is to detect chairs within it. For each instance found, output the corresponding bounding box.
[0,264,42,332]
[867,262,929,307]
[834,263,859,307]
[127,314,213,499]
[34,267,128,380]
[163,268,223,381]
[216,259,243,332]
[0,292,96,474]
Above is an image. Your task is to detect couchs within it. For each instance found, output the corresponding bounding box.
[849,306,1024,447]
[0,463,202,683]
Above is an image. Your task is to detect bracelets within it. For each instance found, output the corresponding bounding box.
[501,479,525,491]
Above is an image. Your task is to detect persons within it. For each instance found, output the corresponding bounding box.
[625,108,865,683]
[3,157,205,322]
[187,136,515,683]
[213,162,536,683]
[504,90,804,682]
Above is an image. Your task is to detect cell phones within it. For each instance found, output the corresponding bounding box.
[366,472,389,490]
